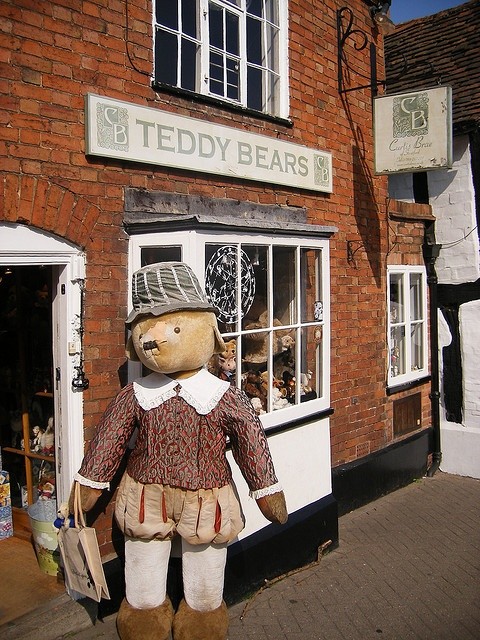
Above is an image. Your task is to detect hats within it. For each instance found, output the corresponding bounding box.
[125,261,217,323]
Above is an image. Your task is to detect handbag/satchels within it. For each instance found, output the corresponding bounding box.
[57,481,111,603]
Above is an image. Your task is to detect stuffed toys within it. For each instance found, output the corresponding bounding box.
[219,357,237,380]
[70,260,288,639]
[314,300,323,320]
[241,310,296,363]
[221,340,238,357]
[58,503,71,530]
[391,347,400,377]
[242,358,316,416]
